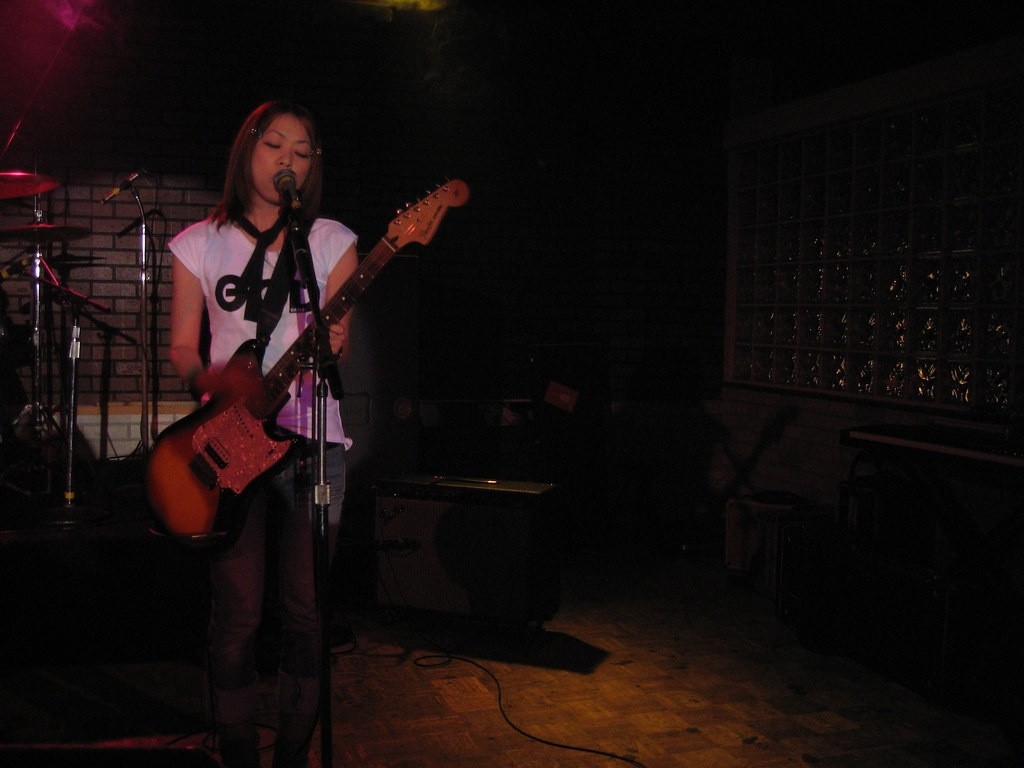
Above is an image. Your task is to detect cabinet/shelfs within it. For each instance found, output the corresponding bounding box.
[728,75,1024,406]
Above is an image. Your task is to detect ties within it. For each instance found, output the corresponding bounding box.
[232,204,291,322]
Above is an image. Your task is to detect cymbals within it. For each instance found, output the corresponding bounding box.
[1,222,91,240]
[0,167,64,199]
[41,253,106,263]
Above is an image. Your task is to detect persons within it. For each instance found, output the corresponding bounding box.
[168,98,357,767]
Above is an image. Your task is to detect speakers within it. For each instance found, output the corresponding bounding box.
[365,474,571,640]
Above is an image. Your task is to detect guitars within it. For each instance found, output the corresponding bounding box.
[143,173,471,546]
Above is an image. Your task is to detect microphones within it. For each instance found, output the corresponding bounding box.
[101,169,146,205]
[273,170,301,210]
